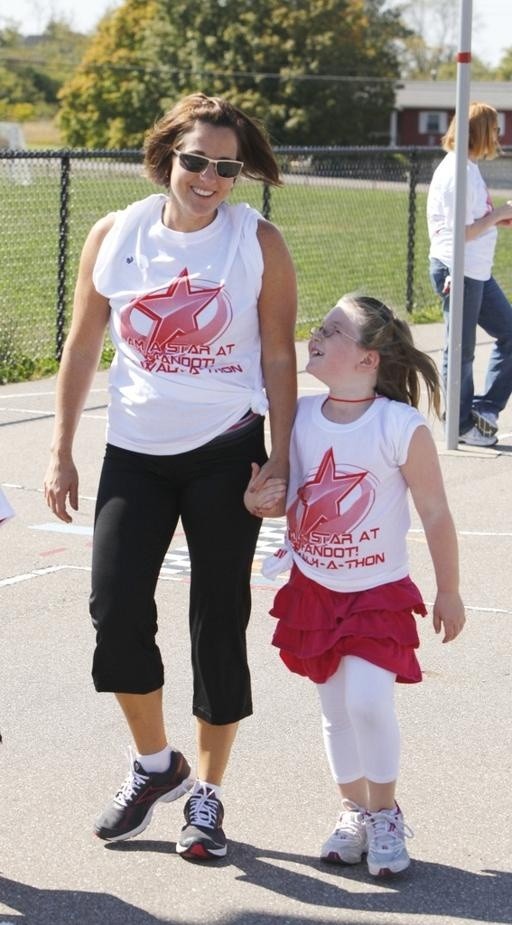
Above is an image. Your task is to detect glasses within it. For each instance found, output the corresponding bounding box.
[307,320,367,346]
[171,147,244,179]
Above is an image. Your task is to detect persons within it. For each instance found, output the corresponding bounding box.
[425,105,512,446]
[245,293,467,879]
[41,94,298,862]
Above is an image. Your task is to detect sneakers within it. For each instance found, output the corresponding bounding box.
[91,751,196,845]
[174,783,229,860]
[468,408,499,438]
[457,425,500,448]
[318,797,368,868]
[363,797,412,876]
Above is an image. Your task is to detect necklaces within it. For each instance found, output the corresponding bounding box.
[327,395,385,405]
